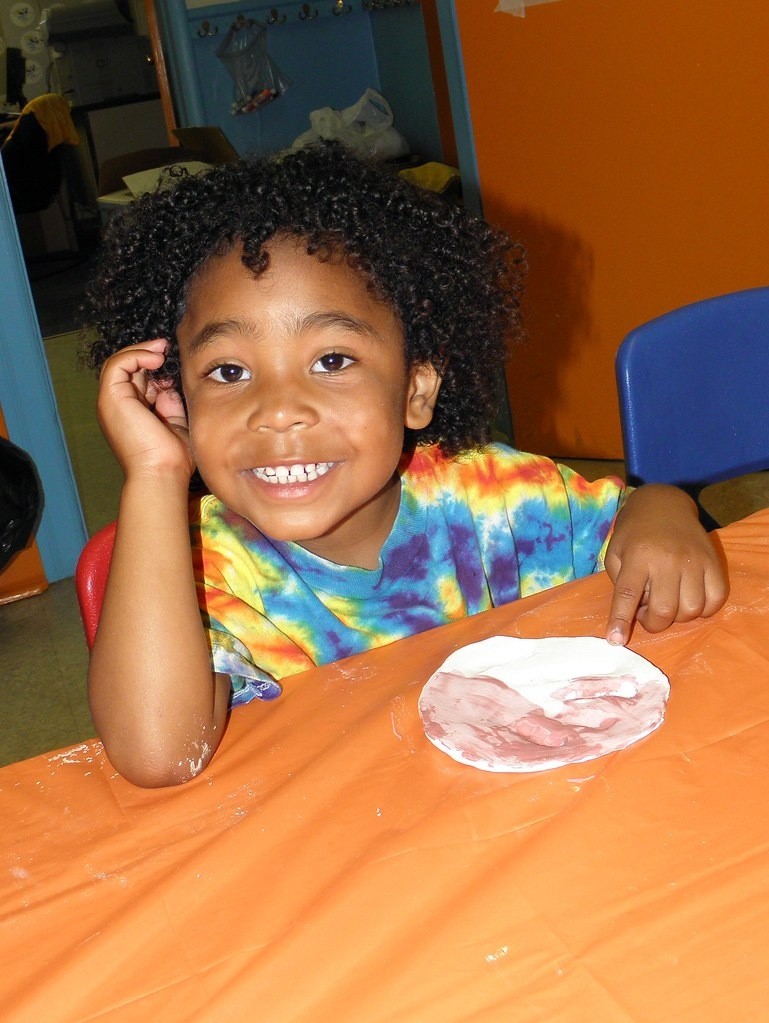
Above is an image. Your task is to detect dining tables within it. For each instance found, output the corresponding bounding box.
[0,502,769,1021]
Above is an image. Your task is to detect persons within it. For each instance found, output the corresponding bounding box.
[85,147,727,789]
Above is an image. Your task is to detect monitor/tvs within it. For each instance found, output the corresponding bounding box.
[0,47,28,114]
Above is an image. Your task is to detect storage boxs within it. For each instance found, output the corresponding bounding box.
[93,125,245,235]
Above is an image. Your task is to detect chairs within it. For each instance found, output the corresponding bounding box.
[1,94,69,215]
[614,285,768,534]
